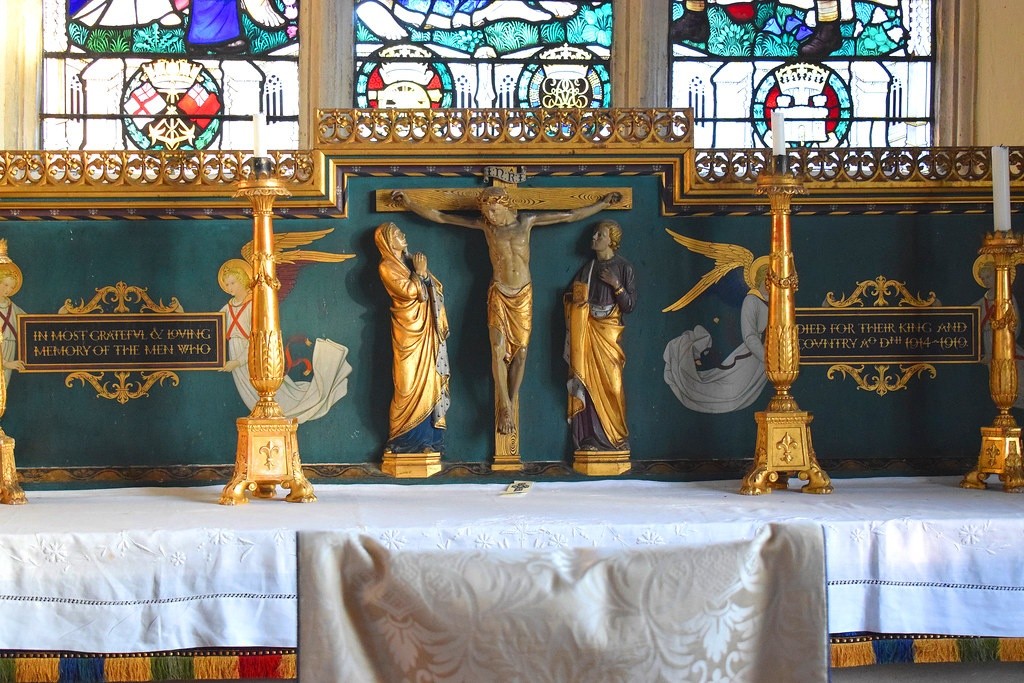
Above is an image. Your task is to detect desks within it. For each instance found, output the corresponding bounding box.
[1,478,1024,683]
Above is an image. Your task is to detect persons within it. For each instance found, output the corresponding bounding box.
[385,182,624,439]
[559,215,636,452]
[372,220,453,453]
